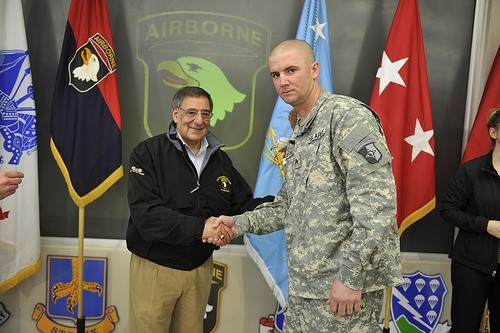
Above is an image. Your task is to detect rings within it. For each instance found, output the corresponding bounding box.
[221,235,224,240]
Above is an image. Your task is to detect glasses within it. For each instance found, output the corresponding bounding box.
[177,106,214,121]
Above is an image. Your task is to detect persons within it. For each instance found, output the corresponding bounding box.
[203,40,404,333]
[0,171,24,200]
[439,108,500,333]
[126,87,276,333]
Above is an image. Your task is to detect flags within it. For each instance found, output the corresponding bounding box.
[48,1,124,207]
[0,0,42,296]
[367,1,437,242]
[241,1,335,317]
